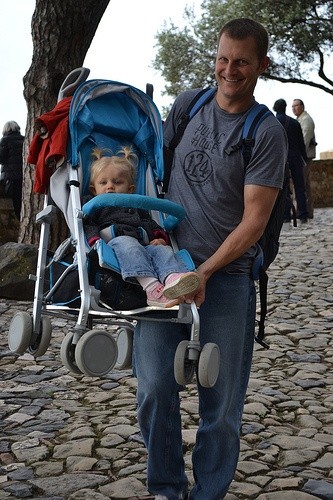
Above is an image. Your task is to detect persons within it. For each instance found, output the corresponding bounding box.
[273,99,307,225]
[85,147,200,308]
[294,99,316,219]
[0,121,25,222]
[135,19,285,500]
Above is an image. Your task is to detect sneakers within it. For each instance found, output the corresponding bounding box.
[147,281,180,307]
[164,272,200,300]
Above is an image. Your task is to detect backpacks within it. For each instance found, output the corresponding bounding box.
[159,87,296,280]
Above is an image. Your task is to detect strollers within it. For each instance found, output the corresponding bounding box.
[8,67,222,389]
